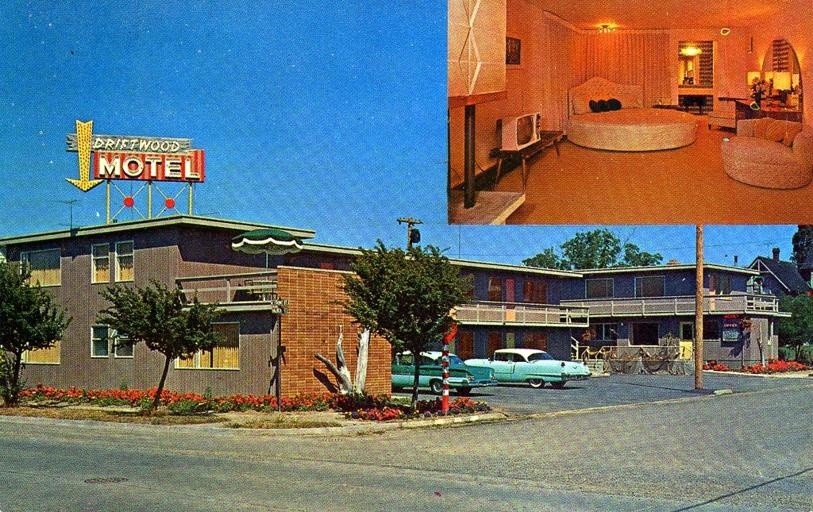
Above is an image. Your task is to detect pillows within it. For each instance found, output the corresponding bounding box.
[571,94,622,114]
[751,118,801,146]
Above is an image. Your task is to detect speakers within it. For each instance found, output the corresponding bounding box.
[411,229,421,243]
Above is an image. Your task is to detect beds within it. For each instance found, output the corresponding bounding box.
[567,76,696,152]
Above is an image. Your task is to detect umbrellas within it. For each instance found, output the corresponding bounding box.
[230,228,304,281]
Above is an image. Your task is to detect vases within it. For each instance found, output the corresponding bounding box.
[754,93,760,108]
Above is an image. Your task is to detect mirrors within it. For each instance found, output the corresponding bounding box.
[676,40,713,88]
[759,39,802,111]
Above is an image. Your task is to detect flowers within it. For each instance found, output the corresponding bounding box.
[749,76,768,96]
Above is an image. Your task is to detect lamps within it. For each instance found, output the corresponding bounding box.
[598,25,615,33]
[771,71,791,108]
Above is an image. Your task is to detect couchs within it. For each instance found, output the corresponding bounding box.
[706,97,747,130]
[721,117,812,189]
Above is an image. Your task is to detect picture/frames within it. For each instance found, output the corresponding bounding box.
[505,32,522,70]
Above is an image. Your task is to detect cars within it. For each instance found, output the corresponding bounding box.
[467,347,590,388]
[391,349,494,394]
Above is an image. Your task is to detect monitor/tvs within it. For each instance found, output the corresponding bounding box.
[498,112,542,150]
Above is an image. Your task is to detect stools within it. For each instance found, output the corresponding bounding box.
[682,95,706,115]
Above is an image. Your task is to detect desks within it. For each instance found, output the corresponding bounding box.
[488,130,563,187]
[734,100,801,137]
